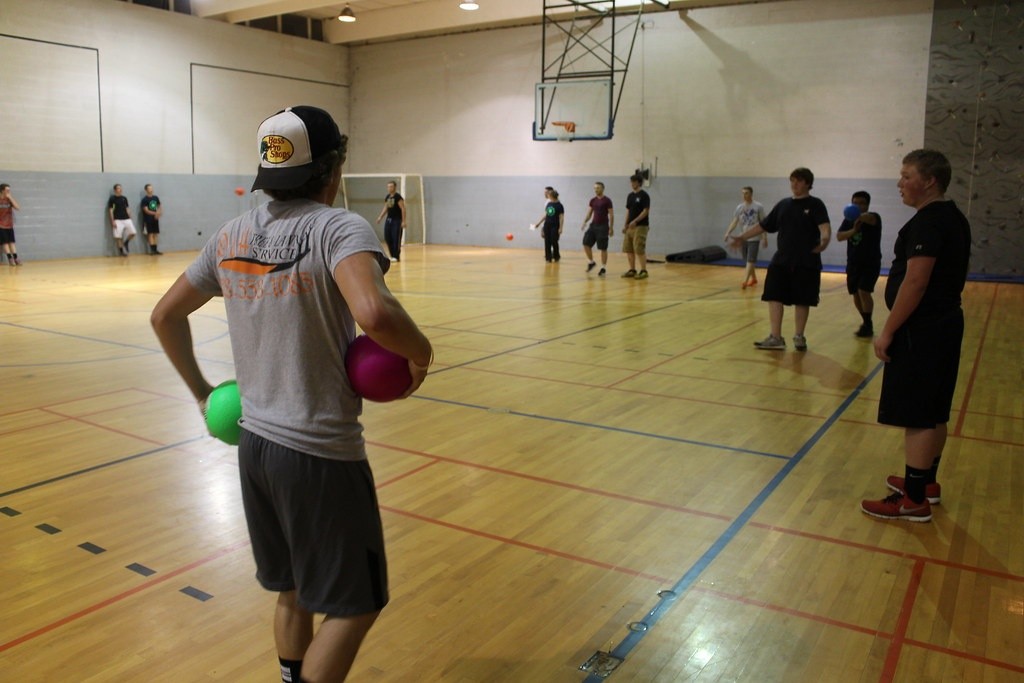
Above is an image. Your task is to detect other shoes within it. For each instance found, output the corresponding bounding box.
[546,258,552,263]
[120,249,127,256]
[10,258,16,266]
[622,270,637,278]
[587,263,596,271]
[598,268,605,275]
[555,255,560,261]
[748,279,757,286]
[150,251,162,256]
[15,259,22,266]
[856,324,873,338]
[634,270,648,279]
[742,282,748,289]
[390,257,398,262]
[124,240,129,252]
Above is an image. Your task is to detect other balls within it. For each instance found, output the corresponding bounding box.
[506,233,513,240]
[235,187,245,196]
[345,333,412,403]
[203,380,242,445]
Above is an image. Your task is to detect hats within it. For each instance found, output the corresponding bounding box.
[250,106,341,193]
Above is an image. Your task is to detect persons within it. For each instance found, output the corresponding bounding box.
[723,186,768,290]
[580,182,614,276]
[726,168,831,352]
[861,149,972,522]
[620,175,651,280]
[376,181,407,263]
[151,104,431,683]
[535,187,564,263]
[0,184,23,266]
[141,184,163,256]
[108,184,136,257]
[837,191,882,338]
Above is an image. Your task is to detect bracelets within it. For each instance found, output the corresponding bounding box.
[411,349,434,368]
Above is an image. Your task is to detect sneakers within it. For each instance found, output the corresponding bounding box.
[861,492,932,521]
[754,333,785,349]
[887,475,940,504]
[793,337,807,349]
